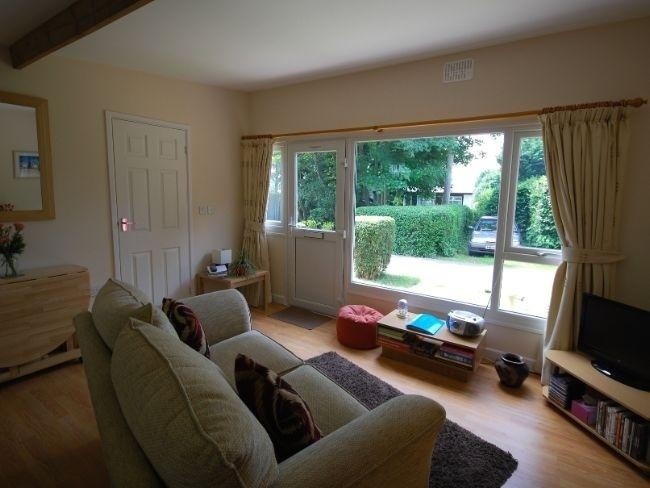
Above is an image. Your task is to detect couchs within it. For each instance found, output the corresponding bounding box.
[71,276,446,485]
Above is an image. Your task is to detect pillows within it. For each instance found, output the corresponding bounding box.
[157,295,211,357]
[231,348,323,461]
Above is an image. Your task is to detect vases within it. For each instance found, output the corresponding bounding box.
[494,352,529,395]
[1,253,18,276]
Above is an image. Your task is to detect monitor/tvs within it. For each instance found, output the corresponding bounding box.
[577,293,650,391]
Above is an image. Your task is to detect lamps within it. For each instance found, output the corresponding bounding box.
[211,248,231,267]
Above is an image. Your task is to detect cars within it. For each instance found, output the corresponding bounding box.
[466,214,525,257]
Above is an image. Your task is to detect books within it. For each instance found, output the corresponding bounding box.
[406,312,445,335]
[376,326,473,369]
[549,372,586,410]
[595,399,648,461]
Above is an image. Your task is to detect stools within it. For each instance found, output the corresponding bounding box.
[337,304,385,348]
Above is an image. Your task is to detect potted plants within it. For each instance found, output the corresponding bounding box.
[226,248,257,281]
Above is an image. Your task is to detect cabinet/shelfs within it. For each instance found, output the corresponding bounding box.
[1,259,91,382]
[374,310,488,380]
[543,348,649,476]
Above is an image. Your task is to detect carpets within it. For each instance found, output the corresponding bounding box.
[267,305,331,330]
[304,352,518,487]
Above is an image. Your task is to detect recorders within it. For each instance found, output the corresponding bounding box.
[446,309,485,338]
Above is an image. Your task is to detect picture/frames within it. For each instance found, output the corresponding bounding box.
[10,148,39,178]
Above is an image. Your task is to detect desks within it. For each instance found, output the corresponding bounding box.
[197,269,270,315]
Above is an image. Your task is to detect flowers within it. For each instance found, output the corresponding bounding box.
[0,219,28,264]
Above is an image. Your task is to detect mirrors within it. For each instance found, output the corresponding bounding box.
[0,89,57,222]
